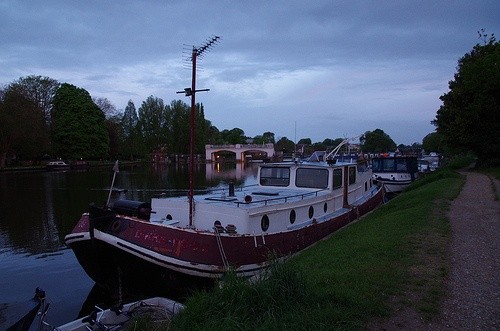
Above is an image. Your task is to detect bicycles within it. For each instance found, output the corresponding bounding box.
[38,297,175,331]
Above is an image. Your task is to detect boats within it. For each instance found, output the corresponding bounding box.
[46,161,68,169]
[64,34,388,287]
[0,285,46,331]
[41,297,186,331]
[369,154,423,194]
